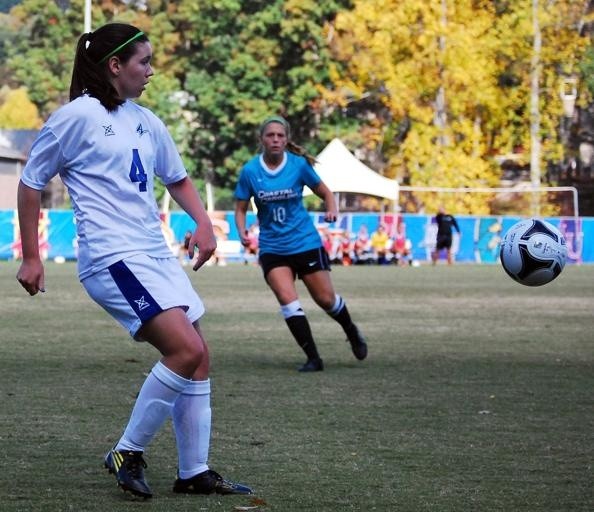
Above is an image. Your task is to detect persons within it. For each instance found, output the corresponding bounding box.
[315,223,413,266]
[16,24,252,497]
[234,118,368,370]
[431,207,460,265]
[243,225,256,264]
[181,231,193,263]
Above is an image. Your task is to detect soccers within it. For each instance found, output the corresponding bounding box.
[499,219,566,286]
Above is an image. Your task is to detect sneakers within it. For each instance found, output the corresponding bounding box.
[102,443,153,495]
[299,354,324,375]
[171,471,254,499]
[346,328,372,363]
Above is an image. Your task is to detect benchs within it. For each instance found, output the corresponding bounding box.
[213,239,242,265]
[329,239,412,261]
